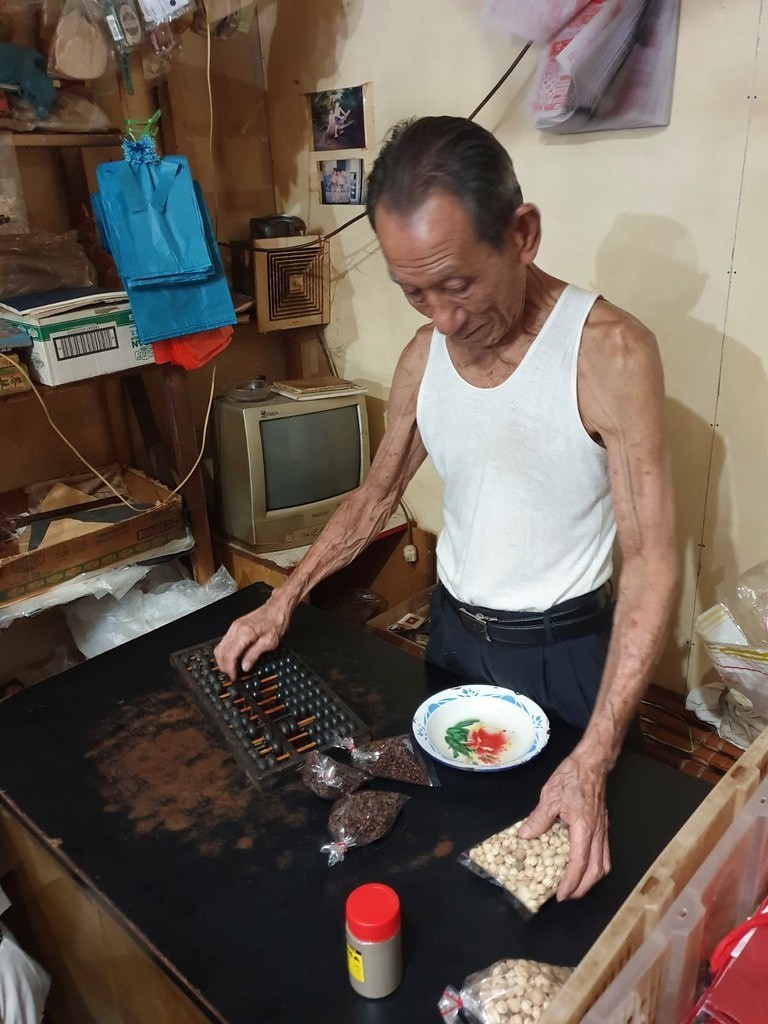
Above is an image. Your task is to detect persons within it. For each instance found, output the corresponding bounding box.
[328,99,351,138]
[325,165,348,204]
[213,116,683,901]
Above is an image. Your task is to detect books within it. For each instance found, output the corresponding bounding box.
[269,374,368,401]
[0,285,130,397]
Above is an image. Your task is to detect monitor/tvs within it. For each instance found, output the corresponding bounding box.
[210,392,371,554]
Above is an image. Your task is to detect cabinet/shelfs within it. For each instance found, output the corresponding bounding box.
[0,43,213,628]
[214,512,416,605]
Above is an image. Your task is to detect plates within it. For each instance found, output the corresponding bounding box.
[412,684,551,772]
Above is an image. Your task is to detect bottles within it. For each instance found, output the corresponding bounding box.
[345,883,403,999]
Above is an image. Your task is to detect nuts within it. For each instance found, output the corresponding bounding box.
[468,817,571,914]
[481,958,575,1024]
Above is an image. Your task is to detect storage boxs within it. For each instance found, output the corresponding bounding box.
[0,463,185,606]
[0,302,155,388]
[364,584,435,659]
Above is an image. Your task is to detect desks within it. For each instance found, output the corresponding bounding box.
[0,582,714,1024]
